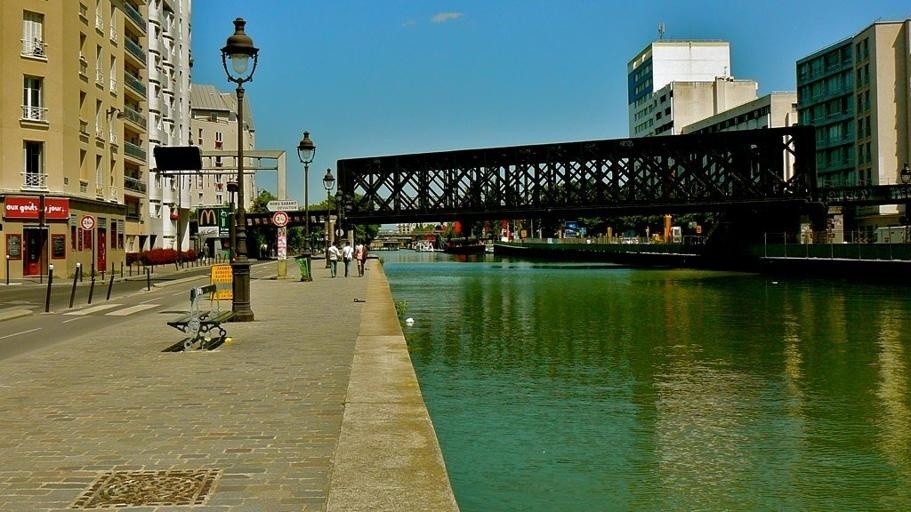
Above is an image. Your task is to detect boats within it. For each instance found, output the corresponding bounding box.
[444,244,487,256]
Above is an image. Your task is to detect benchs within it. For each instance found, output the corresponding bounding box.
[167,283,239,349]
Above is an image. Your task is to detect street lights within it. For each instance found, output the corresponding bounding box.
[297,131,315,281]
[322,169,335,268]
[220,17,260,321]
[335,185,345,249]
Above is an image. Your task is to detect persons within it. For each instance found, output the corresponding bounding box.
[654,231,665,243]
[260,245,266,260]
[342,242,354,277]
[585,236,593,245]
[327,241,341,278]
[353,240,369,277]
[204,242,209,263]
